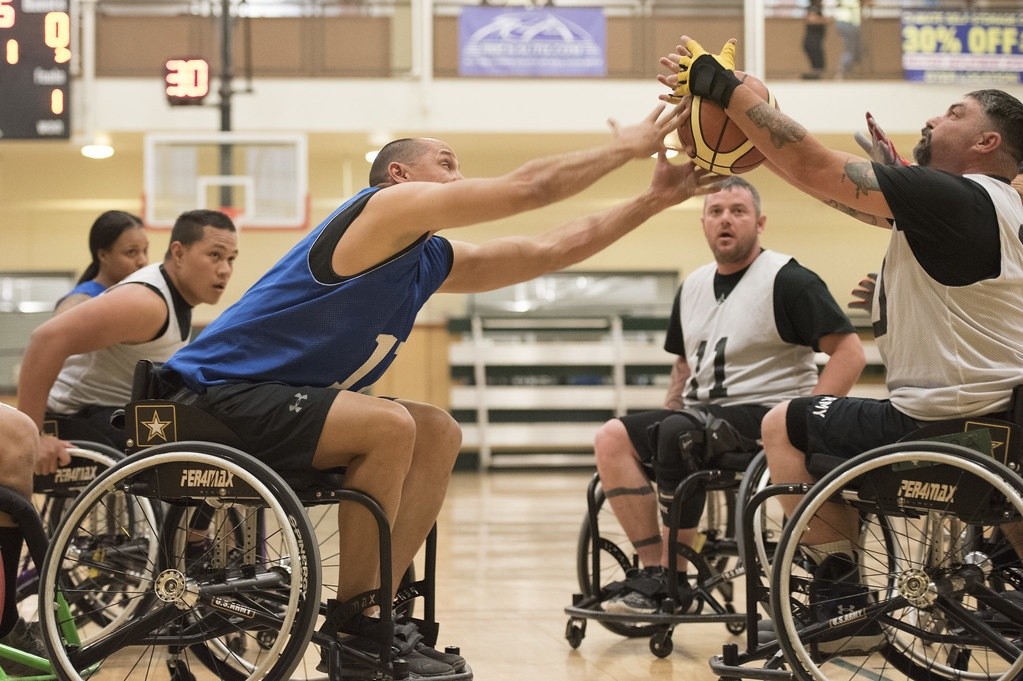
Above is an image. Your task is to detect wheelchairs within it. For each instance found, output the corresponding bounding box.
[708,382,1023,681]
[565,411,903,658]
[0,358,472,681]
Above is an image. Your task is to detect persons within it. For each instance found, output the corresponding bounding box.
[147,97,732,680]
[802,0,876,80]
[596,34,1023,681]
[0,401,52,677]
[18,207,237,576]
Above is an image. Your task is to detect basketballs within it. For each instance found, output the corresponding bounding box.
[677,68,781,176]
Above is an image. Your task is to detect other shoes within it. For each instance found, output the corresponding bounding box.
[600,573,699,615]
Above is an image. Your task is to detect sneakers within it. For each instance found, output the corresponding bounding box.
[316,620,455,677]
[1,617,82,676]
[393,614,466,673]
[759,583,887,656]
